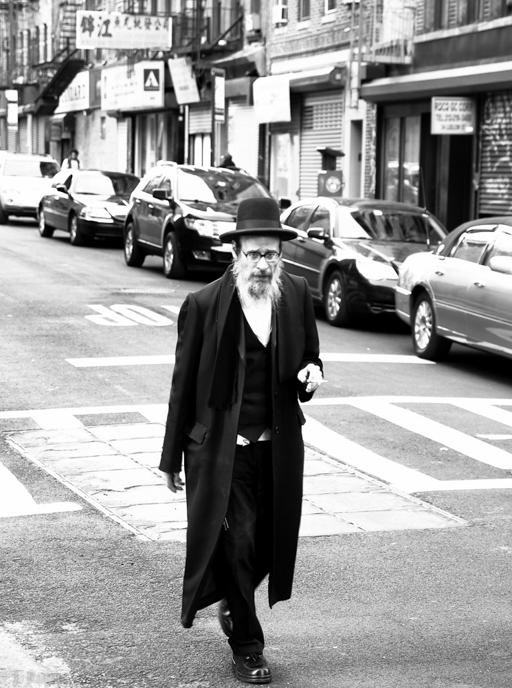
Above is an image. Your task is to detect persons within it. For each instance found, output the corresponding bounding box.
[61,149,81,169]
[216,153,235,169]
[158,199,324,684]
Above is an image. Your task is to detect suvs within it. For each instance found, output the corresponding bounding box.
[123,160,279,279]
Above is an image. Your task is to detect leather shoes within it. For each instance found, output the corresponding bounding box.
[219,599,272,684]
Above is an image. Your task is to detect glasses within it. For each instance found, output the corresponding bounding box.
[238,244,283,262]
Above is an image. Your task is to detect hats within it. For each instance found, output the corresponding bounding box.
[219,198,298,243]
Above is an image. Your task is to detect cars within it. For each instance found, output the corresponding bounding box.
[394,216,512,360]
[36,168,140,247]
[280,195,451,328]
[0,151,63,225]
